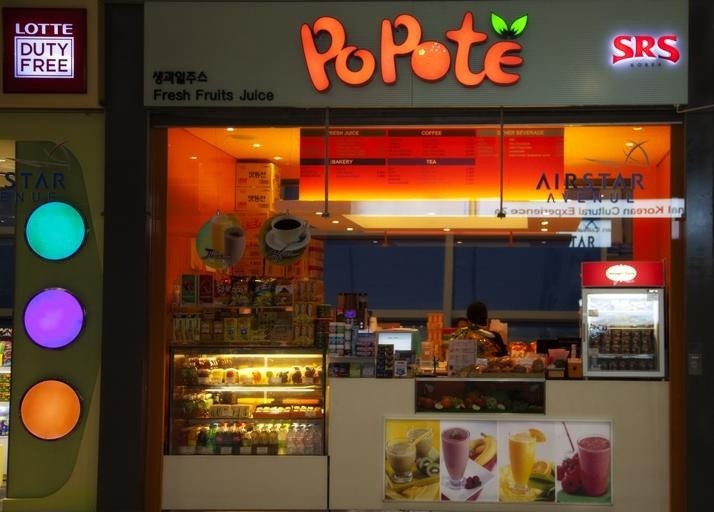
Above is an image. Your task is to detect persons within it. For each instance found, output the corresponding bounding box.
[449,301,508,357]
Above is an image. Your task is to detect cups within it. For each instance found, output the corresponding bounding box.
[196,368,321,387]
[220,228,245,261]
[272,215,307,242]
[209,217,233,253]
[577,433,612,497]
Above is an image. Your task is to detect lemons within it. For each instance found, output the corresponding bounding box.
[529,427,547,443]
[531,460,551,476]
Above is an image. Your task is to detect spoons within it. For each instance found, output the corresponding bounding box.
[278,232,307,255]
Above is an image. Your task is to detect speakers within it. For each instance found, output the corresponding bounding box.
[280,180,299,201]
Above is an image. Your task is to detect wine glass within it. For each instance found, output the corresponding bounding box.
[441,426,473,489]
[406,427,434,465]
[386,436,413,482]
[506,430,539,493]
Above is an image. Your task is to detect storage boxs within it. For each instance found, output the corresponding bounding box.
[221,161,325,285]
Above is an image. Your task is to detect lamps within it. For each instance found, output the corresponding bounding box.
[320,106,331,219]
[496,106,507,220]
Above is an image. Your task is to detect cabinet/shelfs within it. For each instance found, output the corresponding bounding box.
[159,345,330,511]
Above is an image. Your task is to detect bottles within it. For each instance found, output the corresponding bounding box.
[570,344,577,359]
[328,321,351,356]
[177,419,323,457]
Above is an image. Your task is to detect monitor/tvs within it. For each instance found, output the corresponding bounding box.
[375,330,416,352]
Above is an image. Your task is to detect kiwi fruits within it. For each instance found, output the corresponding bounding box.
[418,456,440,479]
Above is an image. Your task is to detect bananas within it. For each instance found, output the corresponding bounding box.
[469,431,497,467]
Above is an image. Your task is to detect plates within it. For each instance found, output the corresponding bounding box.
[265,228,311,253]
[440,453,496,501]
